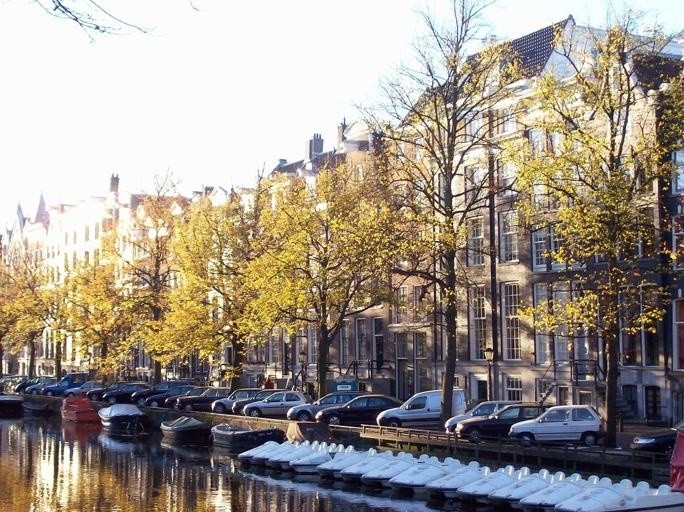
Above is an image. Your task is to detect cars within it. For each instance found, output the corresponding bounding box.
[441,400,522,436]
[628,422,683,462]
[454,400,558,447]
[505,404,606,448]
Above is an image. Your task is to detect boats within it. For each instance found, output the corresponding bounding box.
[159,436,212,466]
[158,415,210,444]
[210,421,287,450]
[92,428,146,456]
[208,443,243,467]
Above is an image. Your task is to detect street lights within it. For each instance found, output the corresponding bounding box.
[298,350,307,390]
[482,348,495,401]
[220,363,227,387]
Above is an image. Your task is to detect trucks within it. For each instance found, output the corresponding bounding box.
[376,387,468,430]
